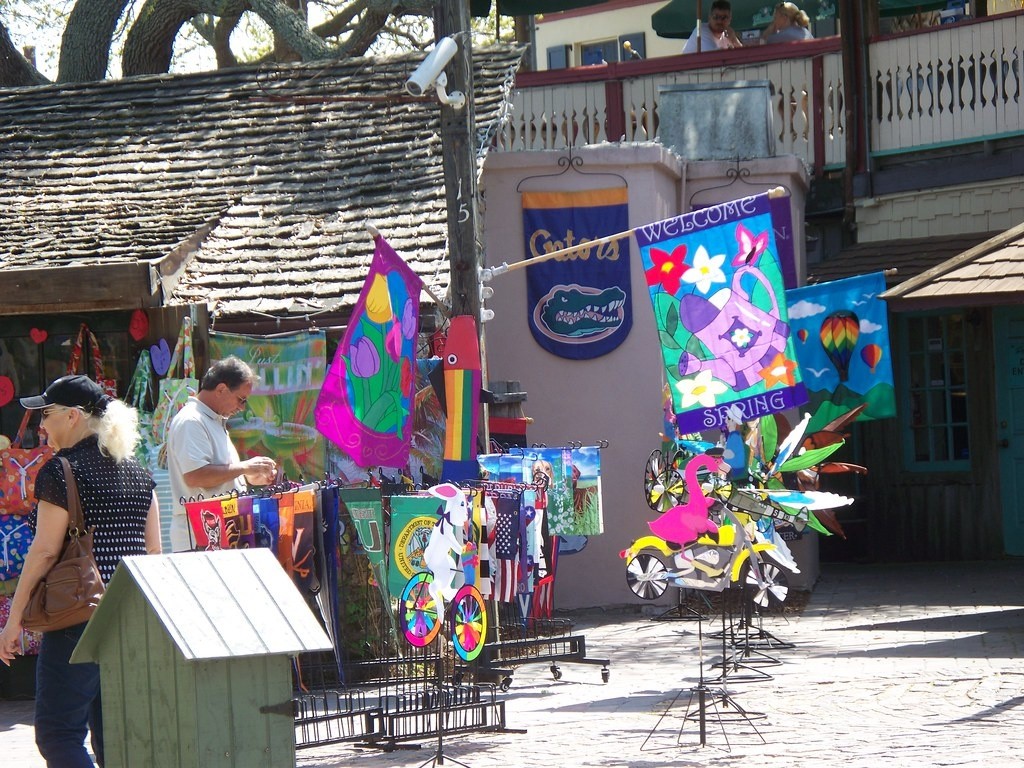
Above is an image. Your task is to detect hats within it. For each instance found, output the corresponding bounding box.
[20,374,106,415]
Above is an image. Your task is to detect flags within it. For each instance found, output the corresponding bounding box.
[315,233,423,469]
[523,186,633,361]
[783,272,897,435]
[692,196,798,291]
[636,192,808,437]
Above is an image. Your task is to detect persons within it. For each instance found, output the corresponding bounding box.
[0,374,162,768]
[166,354,279,554]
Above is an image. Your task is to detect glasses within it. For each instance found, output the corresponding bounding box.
[232,393,248,404]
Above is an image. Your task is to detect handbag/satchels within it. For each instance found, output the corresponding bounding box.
[148,315,201,440]
[62,325,117,397]
[0,408,56,513]
[0,515,34,580]
[21,532,105,630]
[121,349,156,465]
